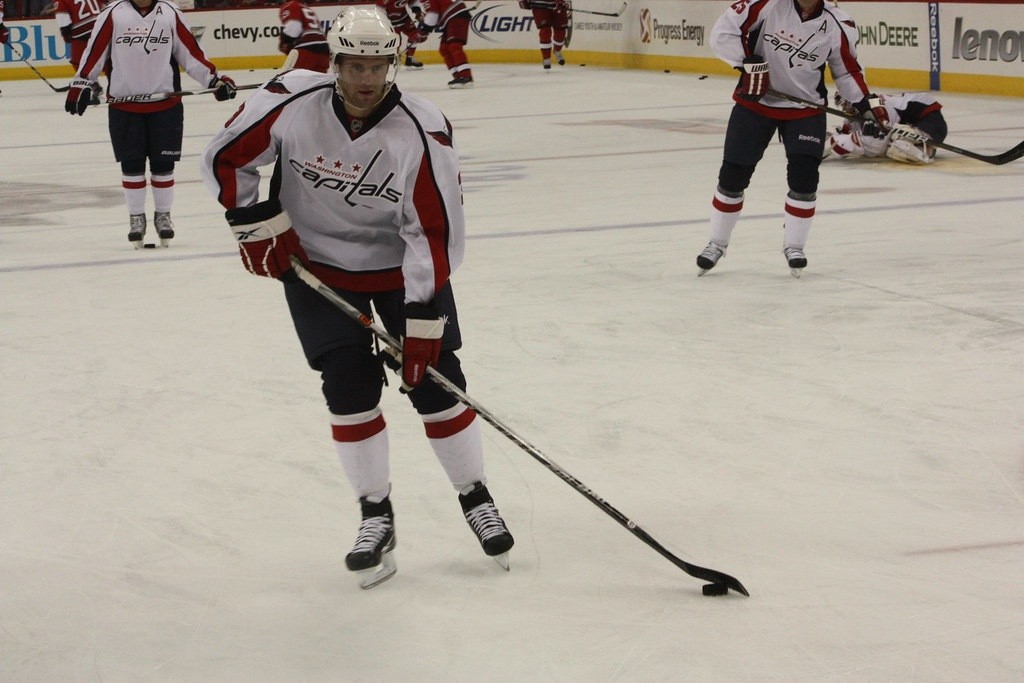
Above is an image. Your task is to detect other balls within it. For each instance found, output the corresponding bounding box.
[250,70,254,72]
[273,67,278,69]
[702,583,729,596]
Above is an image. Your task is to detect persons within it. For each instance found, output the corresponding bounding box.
[0,0,375,106]
[780,88,947,166]
[65,0,237,247]
[695,0,893,280]
[517,0,568,73]
[200,7,515,591]
[419,0,477,89]
[383,0,425,70]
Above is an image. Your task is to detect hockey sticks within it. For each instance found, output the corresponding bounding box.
[401,42,417,54]
[527,2,628,17]
[467,0,482,11]
[88,48,300,107]
[292,260,754,598]
[766,88,1024,166]
[6,42,70,93]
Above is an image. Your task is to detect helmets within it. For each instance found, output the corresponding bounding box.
[834,89,855,113]
[326,4,400,70]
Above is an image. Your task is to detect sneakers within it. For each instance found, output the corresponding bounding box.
[154,211,175,248]
[553,50,565,65]
[127,213,147,250]
[90,82,104,99]
[544,57,551,70]
[458,481,514,572]
[448,76,474,89]
[782,243,807,278]
[696,241,728,277]
[344,482,397,590]
[404,58,424,71]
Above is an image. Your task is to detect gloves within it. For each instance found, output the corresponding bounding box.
[733,55,770,103]
[225,199,310,283]
[65,78,94,115]
[852,93,891,140]
[60,23,73,43]
[415,26,428,43]
[0,23,9,44]
[381,296,445,395]
[553,0,564,16]
[279,41,293,56]
[208,75,237,101]
[519,0,529,9]
